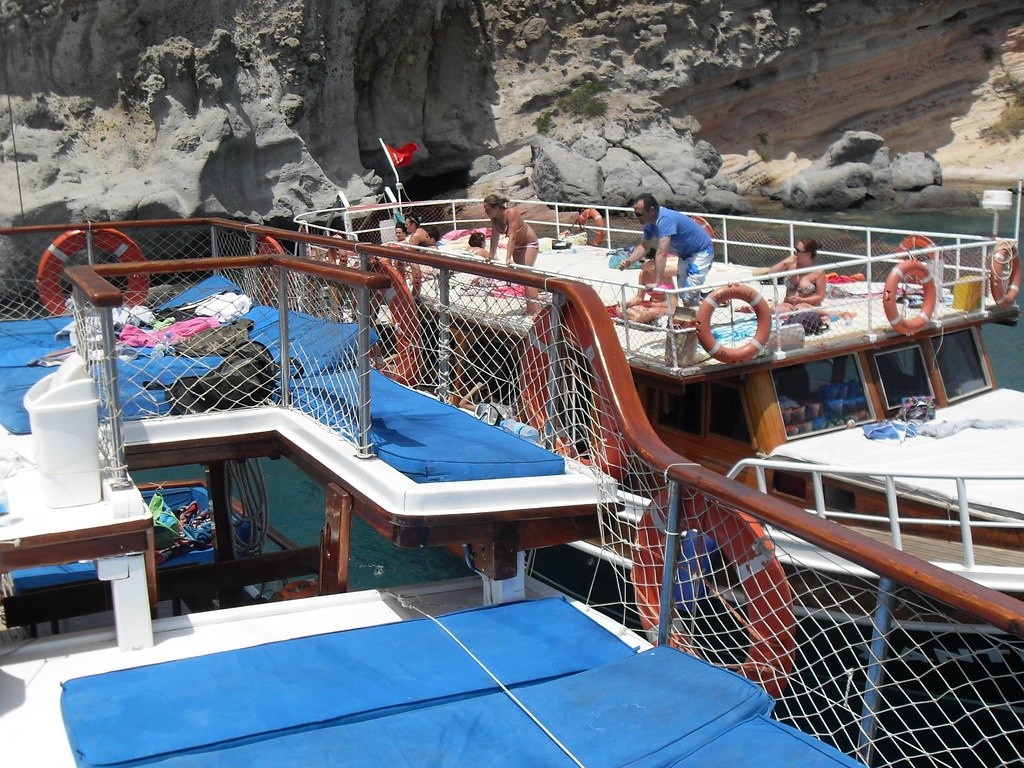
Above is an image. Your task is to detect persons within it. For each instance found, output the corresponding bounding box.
[617,248,679,324]
[466,232,497,259]
[484,195,539,315]
[391,216,444,280]
[770,238,855,322]
[619,193,715,306]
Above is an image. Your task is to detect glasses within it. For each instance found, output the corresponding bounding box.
[634,211,648,217]
[795,246,808,254]
[639,259,652,266]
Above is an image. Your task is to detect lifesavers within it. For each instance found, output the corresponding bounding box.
[895,235,941,267]
[689,215,714,237]
[989,241,1022,308]
[625,477,797,704]
[695,283,774,365]
[882,258,938,337]
[517,295,635,483]
[255,234,287,305]
[351,258,423,388]
[35,225,150,315]
[576,209,607,247]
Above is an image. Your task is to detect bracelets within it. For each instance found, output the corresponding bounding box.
[783,260,786,266]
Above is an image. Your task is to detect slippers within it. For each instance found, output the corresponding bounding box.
[718,299,728,307]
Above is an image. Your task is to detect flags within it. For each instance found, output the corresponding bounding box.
[386,143,419,167]
